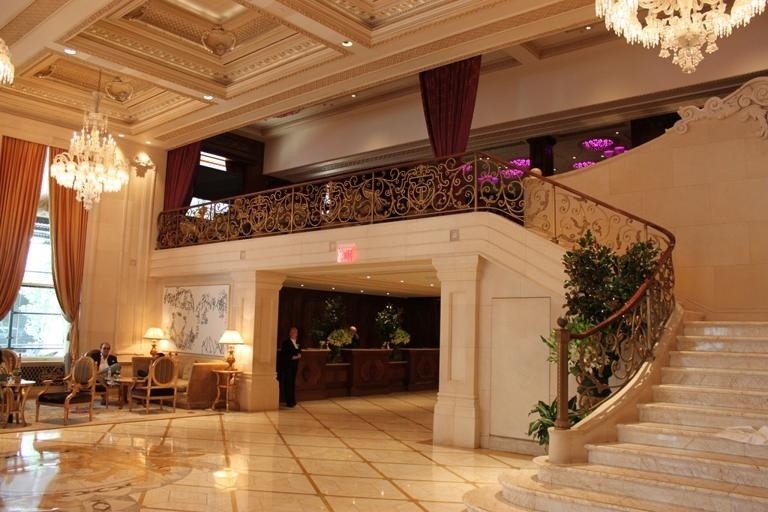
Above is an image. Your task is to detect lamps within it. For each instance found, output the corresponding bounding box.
[218,331,245,371]
[0,37,15,85]
[144,327,165,358]
[50,72,130,210]
[594,0,766,74]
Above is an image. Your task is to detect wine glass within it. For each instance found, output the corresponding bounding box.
[115,368,121,380]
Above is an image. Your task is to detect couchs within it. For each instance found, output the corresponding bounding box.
[163,353,230,410]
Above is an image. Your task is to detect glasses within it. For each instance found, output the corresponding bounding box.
[102,347,111,349]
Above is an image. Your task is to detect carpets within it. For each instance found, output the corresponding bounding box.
[0,398,224,434]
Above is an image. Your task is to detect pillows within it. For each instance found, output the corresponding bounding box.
[172,356,183,378]
[182,358,200,380]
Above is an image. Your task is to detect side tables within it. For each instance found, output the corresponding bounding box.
[212,369,243,412]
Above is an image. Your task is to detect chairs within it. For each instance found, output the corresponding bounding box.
[0,347,178,426]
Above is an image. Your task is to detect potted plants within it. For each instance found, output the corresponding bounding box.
[528,394,582,455]
[562,229,664,420]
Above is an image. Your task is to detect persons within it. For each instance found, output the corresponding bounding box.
[137,353,173,396]
[90,342,117,405]
[282,326,302,407]
[343,326,360,348]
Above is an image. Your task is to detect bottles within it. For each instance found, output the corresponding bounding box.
[107,368,112,378]
[12,354,21,384]
